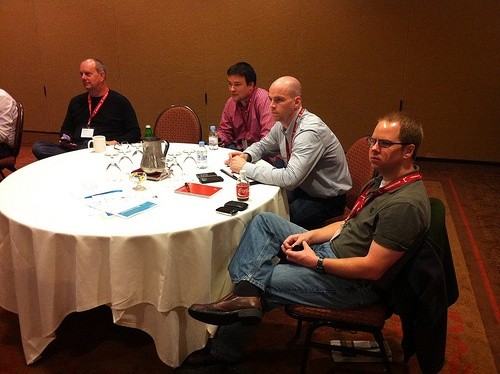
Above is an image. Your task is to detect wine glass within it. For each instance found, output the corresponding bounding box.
[106,142,196,173]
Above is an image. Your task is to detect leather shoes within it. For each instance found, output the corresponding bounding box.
[183,348,240,368]
[188,292,262,326]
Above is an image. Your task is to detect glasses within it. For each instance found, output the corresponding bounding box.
[366,137,407,149]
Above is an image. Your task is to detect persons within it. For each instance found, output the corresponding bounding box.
[215,61,286,168]
[31,57,142,160]
[226,75,352,229]
[185,112,431,369]
[0,88,18,159]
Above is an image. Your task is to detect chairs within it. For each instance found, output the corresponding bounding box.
[0,101,24,180]
[154,105,202,145]
[314,137,378,233]
[286,195,446,374]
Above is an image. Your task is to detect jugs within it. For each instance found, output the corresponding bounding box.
[140,137,169,174]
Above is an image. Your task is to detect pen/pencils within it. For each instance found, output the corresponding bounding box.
[185,183,190,192]
[220,168,237,180]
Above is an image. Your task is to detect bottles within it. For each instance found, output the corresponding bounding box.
[197,141,208,168]
[144,125,152,140]
[208,126,219,150]
[236,169,250,202]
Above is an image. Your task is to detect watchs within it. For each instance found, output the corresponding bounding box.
[316,258,325,275]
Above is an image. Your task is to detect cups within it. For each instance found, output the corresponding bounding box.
[87,136,106,154]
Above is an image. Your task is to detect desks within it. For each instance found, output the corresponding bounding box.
[0,142,290,368]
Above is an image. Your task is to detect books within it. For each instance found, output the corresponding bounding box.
[175,182,223,198]
[221,167,262,184]
